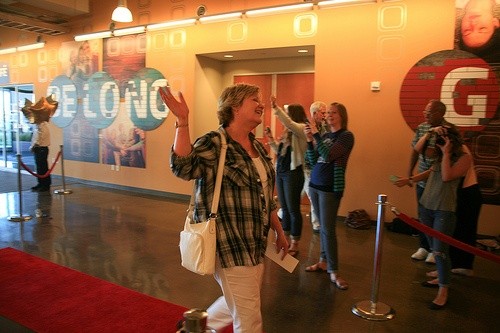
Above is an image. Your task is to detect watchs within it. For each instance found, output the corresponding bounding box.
[175,122,188,128]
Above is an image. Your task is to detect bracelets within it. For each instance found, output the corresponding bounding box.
[409,177,414,187]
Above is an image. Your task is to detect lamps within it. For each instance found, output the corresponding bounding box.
[112,0,133,22]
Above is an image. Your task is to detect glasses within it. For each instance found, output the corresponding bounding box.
[423,111,438,117]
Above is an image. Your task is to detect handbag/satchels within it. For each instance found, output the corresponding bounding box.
[180,216,216,276]
[345,209,370,229]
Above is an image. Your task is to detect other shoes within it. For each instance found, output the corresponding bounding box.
[421,280,438,288]
[277,208,283,219]
[31,183,50,191]
[305,262,328,272]
[178,320,186,333]
[430,298,447,311]
[451,268,474,278]
[331,275,348,289]
[313,223,320,233]
[426,270,438,278]
[289,242,299,257]
[425,253,436,264]
[411,248,428,260]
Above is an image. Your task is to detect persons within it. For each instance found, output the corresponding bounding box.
[263,95,481,310]
[103,124,145,168]
[454,0,500,58]
[159,83,289,333]
[29,121,53,192]
[67,41,99,78]
